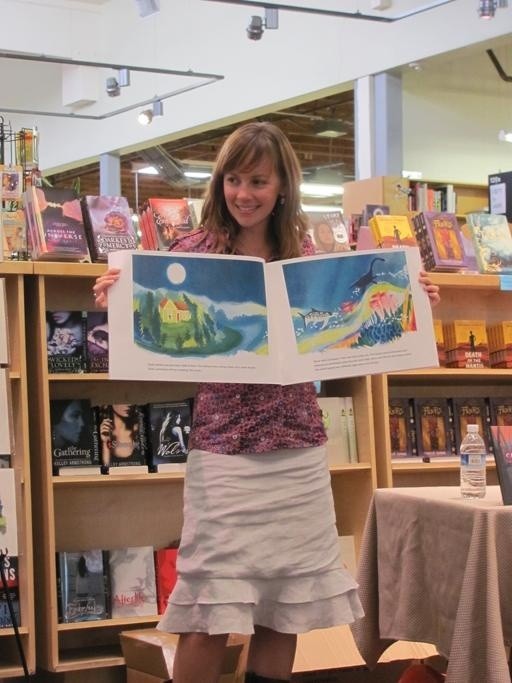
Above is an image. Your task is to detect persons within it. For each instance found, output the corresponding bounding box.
[98,404,145,467]
[159,410,188,456]
[75,556,92,609]
[52,401,84,450]
[92,121,440,683]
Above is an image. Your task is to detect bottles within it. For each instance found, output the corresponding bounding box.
[459,425,486,499]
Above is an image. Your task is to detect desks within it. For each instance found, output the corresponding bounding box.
[346,485,512,683]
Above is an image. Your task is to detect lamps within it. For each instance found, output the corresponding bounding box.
[140,0,159,18]
[247,9,280,41]
[478,0,508,22]
[137,101,165,128]
[106,69,130,99]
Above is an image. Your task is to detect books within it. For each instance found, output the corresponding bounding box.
[155,549,178,615]
[94,403,148,467]
[301,208,414,270]
[43,310,109,374]
[0,187,93,262]
[434,318,512,369]
[54,549,107,624]
[58,466,101,475]
[415,205,512,274]
[488,424,512,505]
[388,396,512,458]
[148,403,195,466]
[106,245,440,384]
[103,545,159,619]
[108,466,147,474]
[407,182,456,214]
[82,192,206,264]
[315,396,358,464]
[49,397,93,465]
[157,462,188,474]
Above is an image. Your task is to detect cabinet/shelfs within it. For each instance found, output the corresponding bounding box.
[0,261,35,683]
[34,261,380,673]
[342,176,512,234]
[372,273,511,488]
